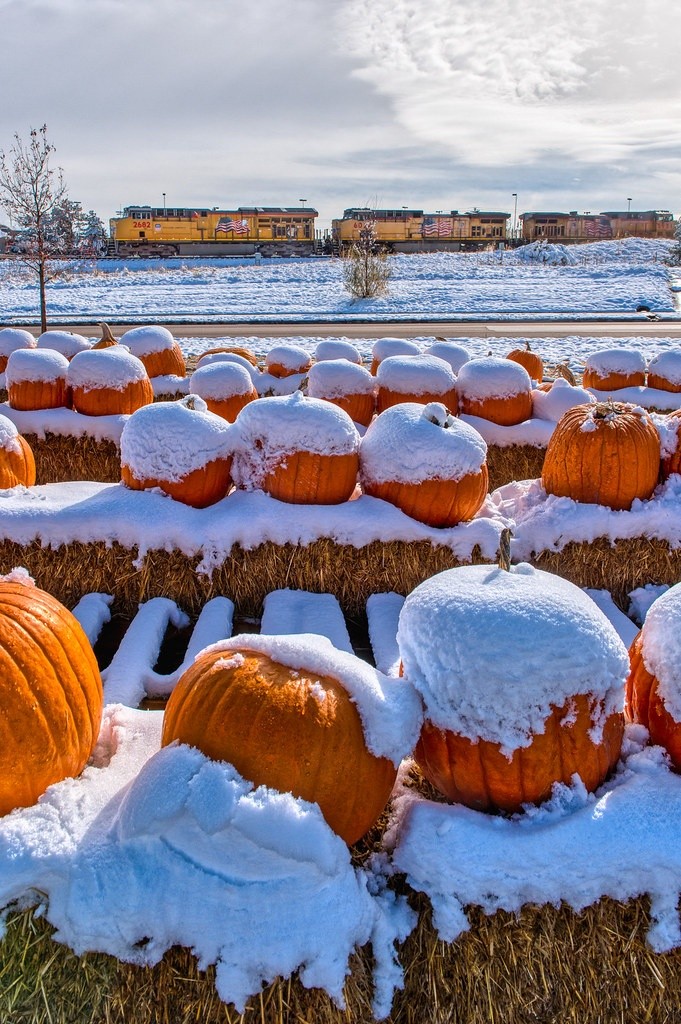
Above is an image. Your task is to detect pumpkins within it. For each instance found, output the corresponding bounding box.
[161,645,403,849]
[0,573,103,818]
[0,319,681,527]
[620,626,681,775]
[398,529,625,813]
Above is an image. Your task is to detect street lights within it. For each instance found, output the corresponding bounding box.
[163,193,166,208]
[299,199,307,208]
[512,194,518,231]
[627,198,632,212]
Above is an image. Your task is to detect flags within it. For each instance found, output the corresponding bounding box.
[216,217,251,233]
[418,219,452,234]
[585,221,611,237]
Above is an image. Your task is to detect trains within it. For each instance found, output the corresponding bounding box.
[109,205,675,261]
